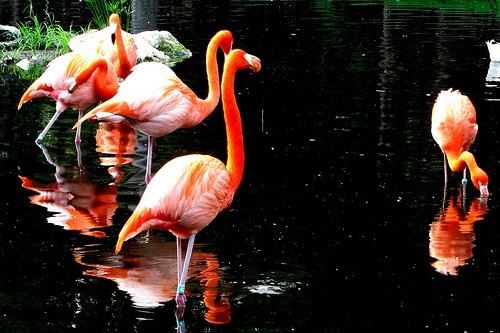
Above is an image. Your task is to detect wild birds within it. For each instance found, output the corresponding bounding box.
[429,183,487,275]
[72,30,233,185]
[19,121,232,324]
[114,49,261,307]
[430,88,489,196]
[18,13,137,142]
